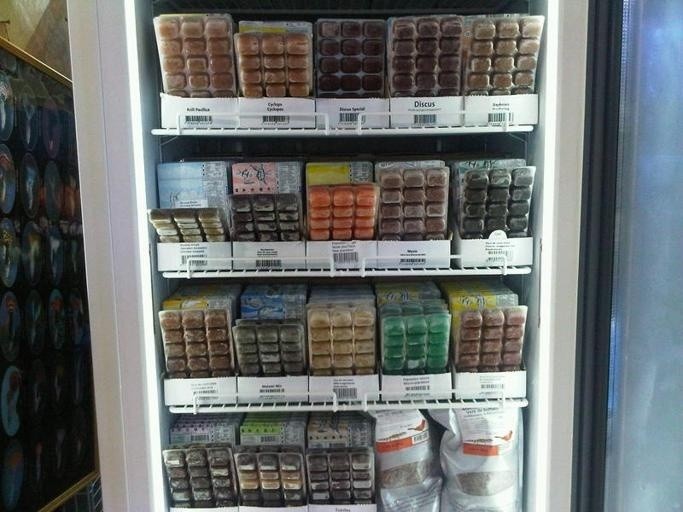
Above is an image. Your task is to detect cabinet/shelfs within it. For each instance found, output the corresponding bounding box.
[65,1,588,512]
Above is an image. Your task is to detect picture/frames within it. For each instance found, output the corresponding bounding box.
[0,36,100,512]
